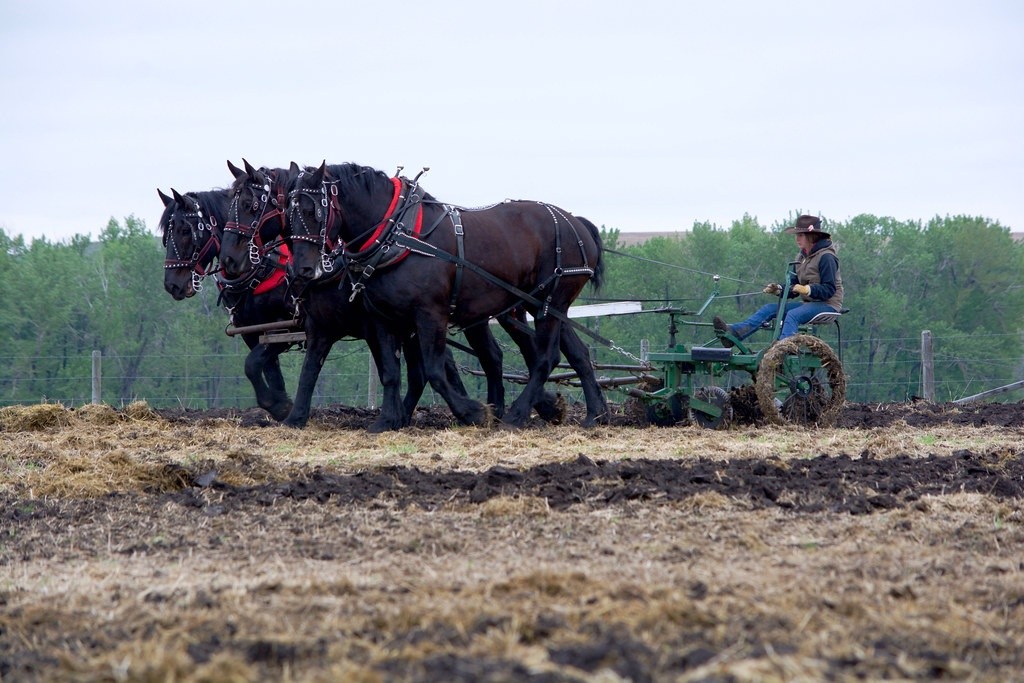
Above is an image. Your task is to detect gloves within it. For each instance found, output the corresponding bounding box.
[763,283,783,296]
[792,284,811,296]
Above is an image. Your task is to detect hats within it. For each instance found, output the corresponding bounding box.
[784,215,831,240]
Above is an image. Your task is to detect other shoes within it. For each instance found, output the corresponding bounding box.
[712,317,735,348]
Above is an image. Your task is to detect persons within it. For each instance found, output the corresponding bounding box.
[712,215,843,348]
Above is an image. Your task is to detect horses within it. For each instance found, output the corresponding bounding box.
[155,155,610,431]
